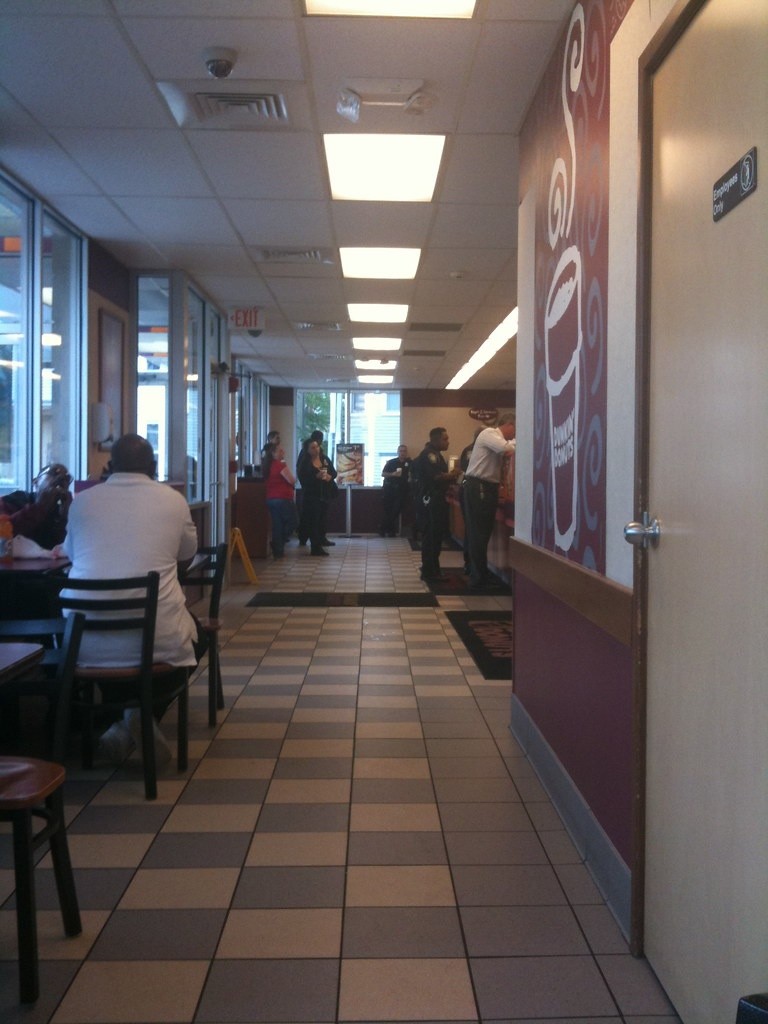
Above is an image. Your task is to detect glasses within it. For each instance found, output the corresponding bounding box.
[37,467,74,485]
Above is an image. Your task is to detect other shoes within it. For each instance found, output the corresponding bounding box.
[310,548,329,556]
[100,720,136,769]
[464,565,493,582]
[322,539,335,545]
[125,701,172,765]
[421,571,448,582]
[272,551,283,561]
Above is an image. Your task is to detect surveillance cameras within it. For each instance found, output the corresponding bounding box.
[247,316,265,336]
[203,47,237,78]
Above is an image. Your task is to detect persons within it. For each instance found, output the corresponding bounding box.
[299,438,338,557]
[460,424,487,473]
[296,429,325,547]
[260,440,298,557]
[265,430,280,447]
[381,443,412,535]
[59,433,199,771]
[461,412,516,582]
[407,441,456,551]
[7,462,73,559]
[417,426,459,582]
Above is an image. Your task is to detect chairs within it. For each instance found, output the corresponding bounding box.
[177,544,225,728]
[0,611,82,1003]
[31,572,190,802]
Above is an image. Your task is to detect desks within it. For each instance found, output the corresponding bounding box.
[0,642,45,687]
[0,554,218,728]
